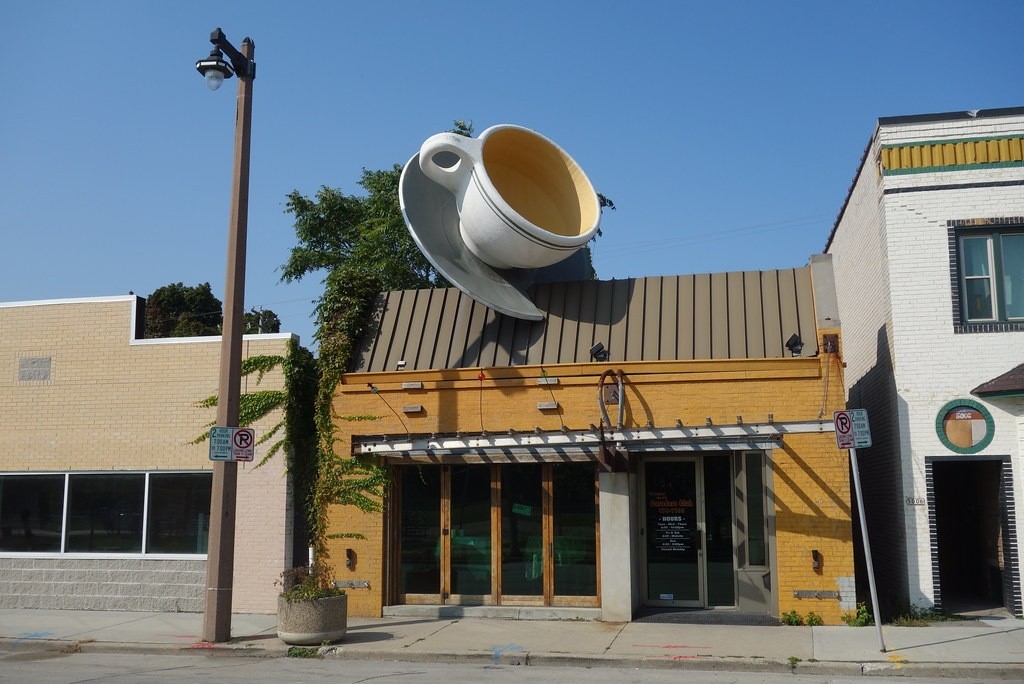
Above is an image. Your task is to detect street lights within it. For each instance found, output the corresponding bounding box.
[192,28,255,643]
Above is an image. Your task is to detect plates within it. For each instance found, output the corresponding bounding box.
[399,151,544,321]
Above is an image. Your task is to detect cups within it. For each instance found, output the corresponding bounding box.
[418,124,600,271]
